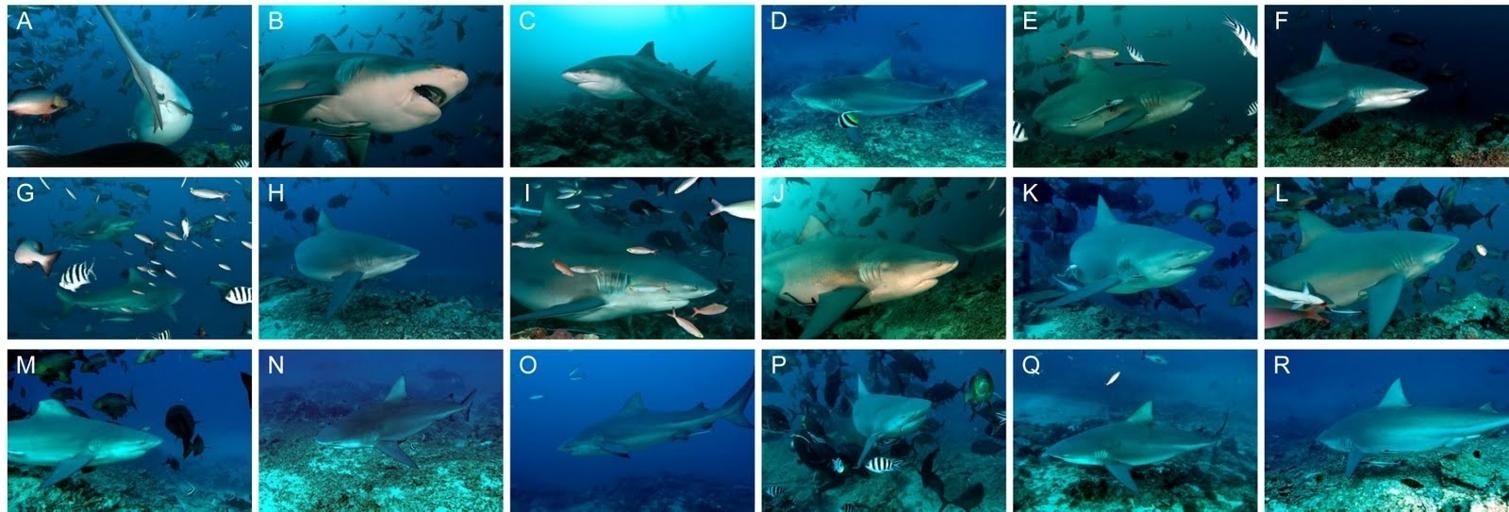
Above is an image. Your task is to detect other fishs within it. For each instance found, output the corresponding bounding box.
[8,350,166,421]
[838,110,865,128]
[706,193,754,220]
[1265,178,1508,330]
[1014,178,1256,319]
[1141,350,1168,367]
[511,177,730,338]
[762,178,1005,256]
[762,350,1006,512]
[165,404,204,472]
[192,350,231,361]
[1105,370,1121,387]
[263,6,503,166]
[529,395,544,400]
[1013,6,1257,144]
[449,210,503,231]
[569,367,582,381]
[9,177,252,340]
[8,5,251,167]
[259,182,351,226]
